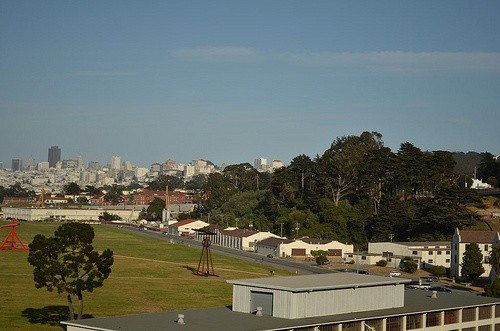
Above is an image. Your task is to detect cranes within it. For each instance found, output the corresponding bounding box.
[36,185,46,209]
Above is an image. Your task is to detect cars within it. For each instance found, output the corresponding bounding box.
[390,271,401,277]
[406,282,419,289]
[356,270,369,275]
[162,232,169,235]
[139,227,148,231]
[427,277,438,282]
[414,284,430,289]
